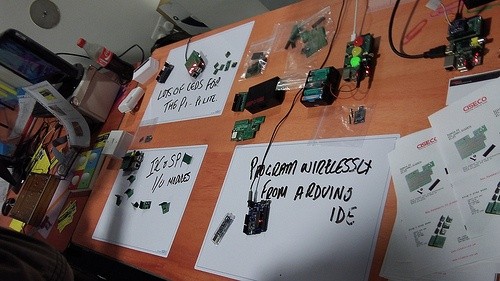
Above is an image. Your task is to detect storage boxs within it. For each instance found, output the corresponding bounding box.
[100,129,135,161]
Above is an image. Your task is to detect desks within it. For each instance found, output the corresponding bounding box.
[69,1,499,280]
[0,64,141,280]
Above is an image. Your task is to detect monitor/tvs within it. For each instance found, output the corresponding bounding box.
[0,29,78,84]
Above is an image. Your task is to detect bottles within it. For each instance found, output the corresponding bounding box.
[76,37,135,82]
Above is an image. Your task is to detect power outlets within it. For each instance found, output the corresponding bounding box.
[150,16,173,43]
